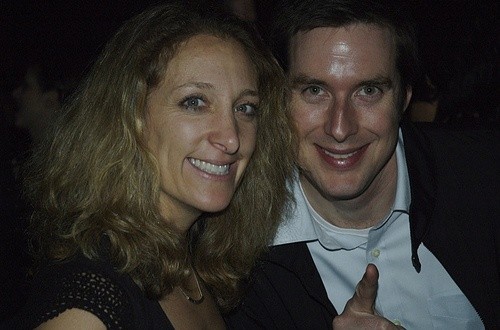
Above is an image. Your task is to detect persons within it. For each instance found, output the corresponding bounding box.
[10,55,79,144]
[17,0,332,330]
[244,0,500,330]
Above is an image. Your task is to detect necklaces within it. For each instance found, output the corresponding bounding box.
[179,263,205,304]
